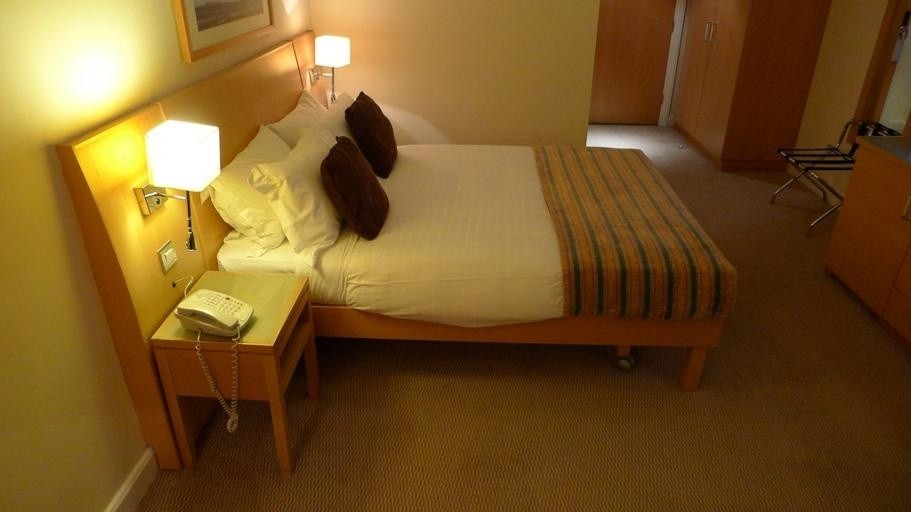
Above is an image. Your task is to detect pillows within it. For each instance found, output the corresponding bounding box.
[320,135,390,240]
[344,91,398,179]
[299,93,355,139]
[246,126,341,268]
[199,125,293,251]
[265,88,328,148]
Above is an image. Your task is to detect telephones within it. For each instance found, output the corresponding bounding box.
[174,289,253,337]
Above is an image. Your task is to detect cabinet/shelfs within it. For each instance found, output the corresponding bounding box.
[673,1,832,172]
[821,136,911,357]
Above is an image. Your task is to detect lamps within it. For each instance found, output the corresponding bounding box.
[133,119,222,252]
[309,34,351,104]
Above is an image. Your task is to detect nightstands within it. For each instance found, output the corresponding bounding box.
[150,270,320,474]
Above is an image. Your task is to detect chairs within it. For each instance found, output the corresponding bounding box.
[771,118,904,238]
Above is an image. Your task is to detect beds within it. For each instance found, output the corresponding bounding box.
[57,28,738,473]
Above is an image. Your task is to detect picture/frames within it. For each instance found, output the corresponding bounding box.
[170,0,282,65]
